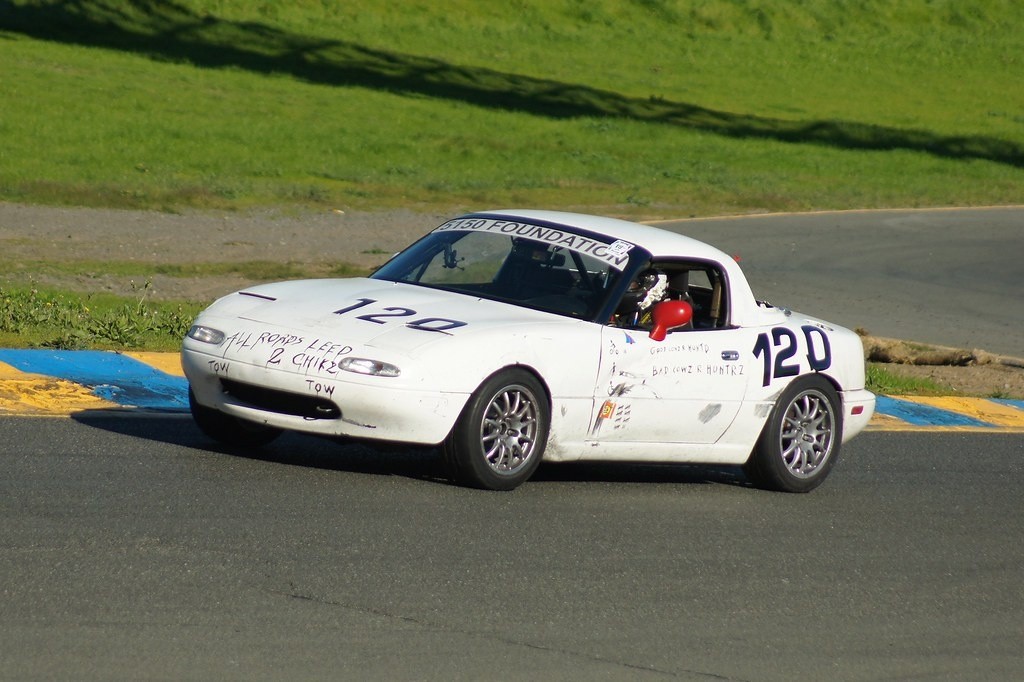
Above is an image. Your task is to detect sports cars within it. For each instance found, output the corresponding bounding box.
[180,208,876,491]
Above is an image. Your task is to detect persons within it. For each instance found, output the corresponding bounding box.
[603,266,670,325]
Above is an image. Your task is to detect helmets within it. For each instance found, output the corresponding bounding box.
[622,268,669,313]
